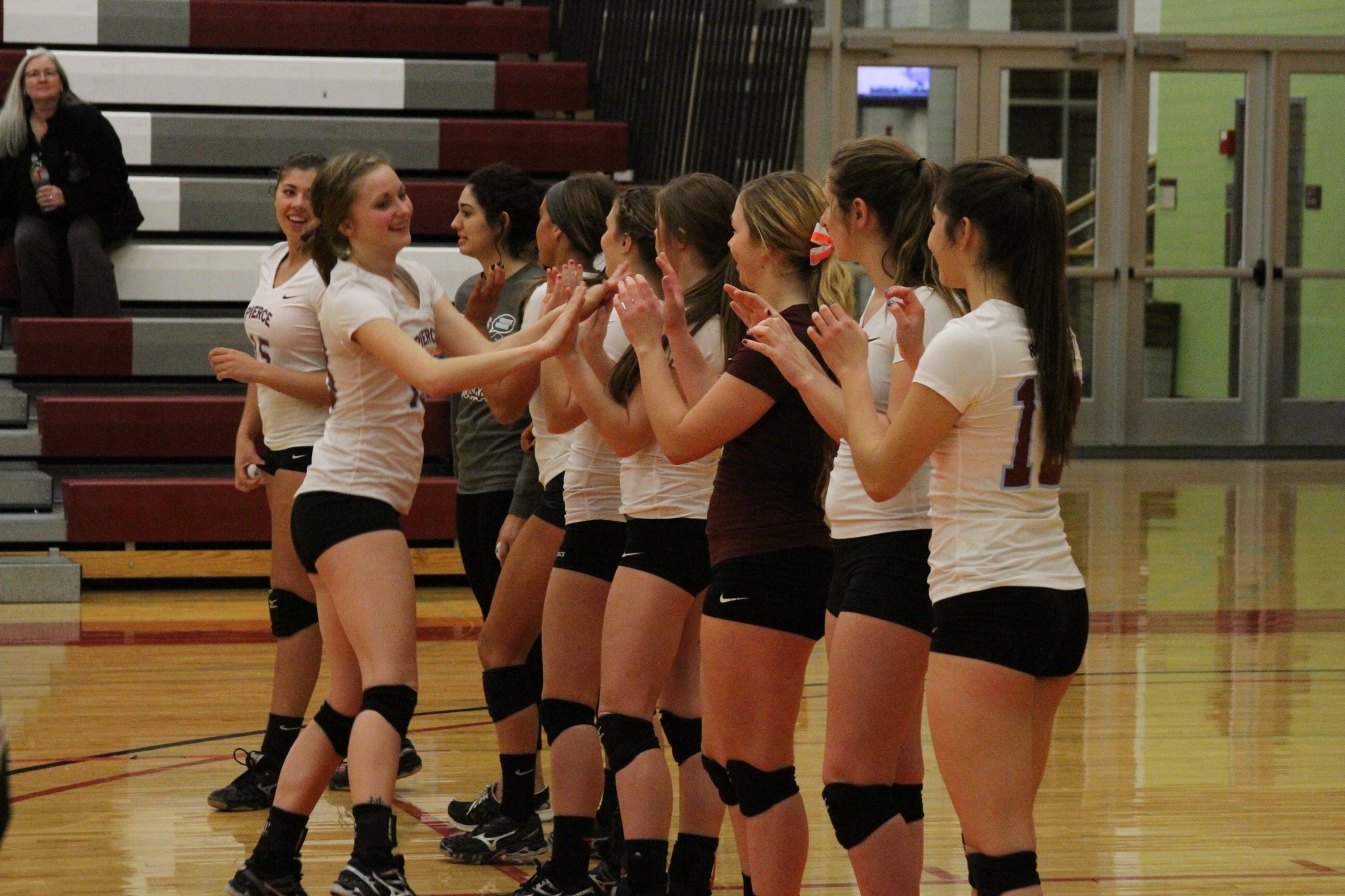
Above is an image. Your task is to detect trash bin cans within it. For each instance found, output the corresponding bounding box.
[1140,299,1179,399]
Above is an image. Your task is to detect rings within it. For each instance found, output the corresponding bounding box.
[50,195,53,199]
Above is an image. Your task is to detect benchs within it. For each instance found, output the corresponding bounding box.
[0,0,667,544]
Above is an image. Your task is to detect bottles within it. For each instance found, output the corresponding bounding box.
[30,153,57,212]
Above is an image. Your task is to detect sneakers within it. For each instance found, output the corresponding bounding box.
[447,781,553,831]
[329,854,414,896]
[330,739,422,789]
[207,751,279,811]
[439,812,549,863]
[227,851,309,896]
[510,858,619,896]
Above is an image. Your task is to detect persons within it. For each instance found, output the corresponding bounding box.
[721,140,970,896]
[209,150,422,810]
[808,155,1087,896]
[225,148,637,896]
[615,172,843,896]
[0,48,144,320]
[432,164,756,896]
